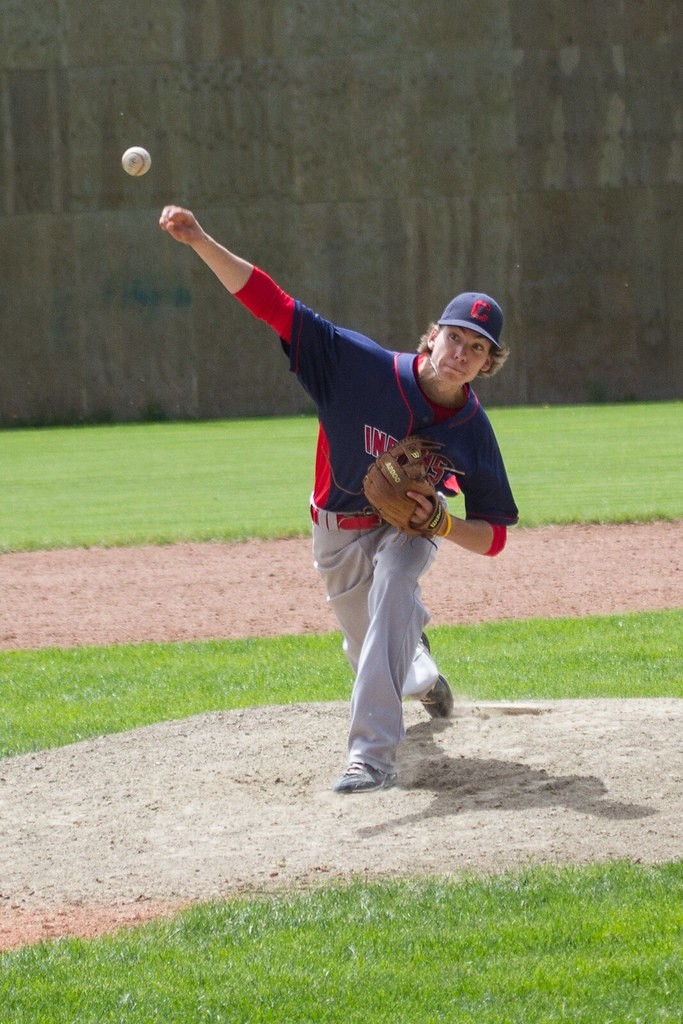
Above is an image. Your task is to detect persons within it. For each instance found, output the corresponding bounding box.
[159,204,520,793]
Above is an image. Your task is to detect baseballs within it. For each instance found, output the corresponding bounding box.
[120,146,152,177]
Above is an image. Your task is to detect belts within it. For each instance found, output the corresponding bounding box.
[312,507,386,531]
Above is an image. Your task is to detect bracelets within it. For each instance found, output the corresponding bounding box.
[437,511,451,538]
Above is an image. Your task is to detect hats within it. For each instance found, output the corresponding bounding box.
[437,292,504,347]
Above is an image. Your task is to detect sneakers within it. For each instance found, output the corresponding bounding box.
[332,762,398,793]
[417,631,453,720]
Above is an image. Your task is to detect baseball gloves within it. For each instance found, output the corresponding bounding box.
[359,435,448,541]
